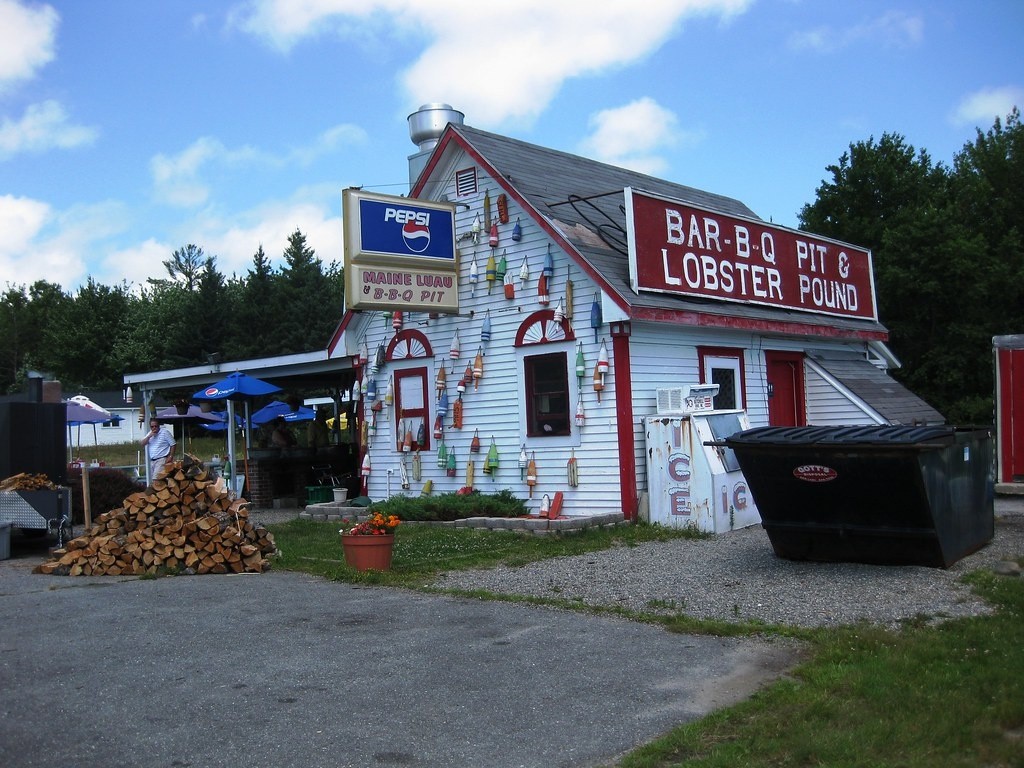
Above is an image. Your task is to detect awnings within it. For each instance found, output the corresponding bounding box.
[244,401,317,448]
[193,372,283,507]
[139,403,227,461]
[197,411,260,461]
[66,395,125,469]
[326,412,358,430]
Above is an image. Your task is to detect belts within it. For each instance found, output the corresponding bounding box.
[151,456,167,461]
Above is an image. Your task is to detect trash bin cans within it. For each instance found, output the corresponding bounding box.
[701,425,998,568]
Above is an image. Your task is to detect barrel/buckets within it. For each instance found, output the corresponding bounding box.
[332,487,348,504]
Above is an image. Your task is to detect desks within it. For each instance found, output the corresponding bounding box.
[110,463,147,487]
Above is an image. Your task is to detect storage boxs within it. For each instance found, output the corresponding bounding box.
[304,483,342,506]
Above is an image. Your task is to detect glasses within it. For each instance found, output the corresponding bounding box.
[150,426,158,428]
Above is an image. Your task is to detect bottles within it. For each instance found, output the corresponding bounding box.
[351,187,610,500]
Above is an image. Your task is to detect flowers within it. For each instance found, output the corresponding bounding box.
[337,510,400,537]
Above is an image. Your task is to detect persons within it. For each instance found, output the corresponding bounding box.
[141,419,176,482]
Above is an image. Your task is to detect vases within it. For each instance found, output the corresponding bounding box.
[332,487,348,503]
[340,534,394,571]
